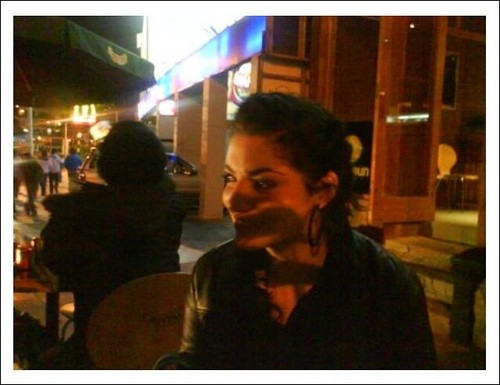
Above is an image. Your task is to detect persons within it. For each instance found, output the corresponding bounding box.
[13,137,83,213]
[176,88,435,370]
[38,118,188,366]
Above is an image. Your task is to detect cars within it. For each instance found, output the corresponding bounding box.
[164,151,197,176]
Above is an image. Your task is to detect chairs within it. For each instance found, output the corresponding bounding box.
[87,268,198,370]
